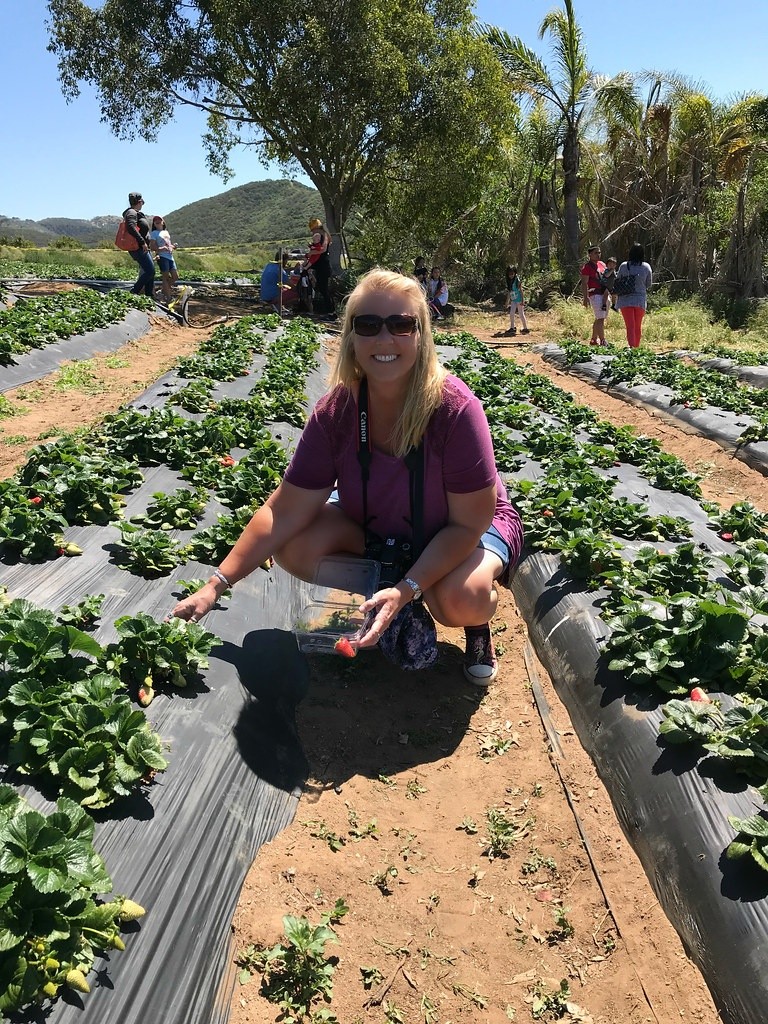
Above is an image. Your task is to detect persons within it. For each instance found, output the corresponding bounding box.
[580,245,611,346]
[503,264,529,334]
[415,269,428,288]
[414,256,424,270]
[301,233,322,270]
[307,219,339,321]
[166,270,526,687]
[612,243,653,348]
[123,192,157,299]
[427,266,448,321]
[151,214,180,303]
[599,257,619,312]
[260,250,300,313]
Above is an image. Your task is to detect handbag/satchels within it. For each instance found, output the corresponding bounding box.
[612,260,639,295]
[115,209,141,251]
[369,577,439,673]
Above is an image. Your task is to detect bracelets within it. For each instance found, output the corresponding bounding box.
[213,570,233,589]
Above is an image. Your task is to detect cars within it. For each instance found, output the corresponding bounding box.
[289,248,309,259]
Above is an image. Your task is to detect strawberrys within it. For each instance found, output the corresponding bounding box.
[543,509,553,518]
[55,542,84,556]
[208,401,219,411]
[28,497,42,508]
[137,686,155,707]
[241,369,250,376]
[691,686,711,706]
[239,442,245,448]
[721,533,732,541]
[331,635,355,660]
[36,899,146,998]
[219,455,235,467]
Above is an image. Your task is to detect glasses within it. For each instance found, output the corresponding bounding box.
[351,314,420,336]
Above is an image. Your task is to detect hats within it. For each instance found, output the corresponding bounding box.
[129,193,142,205]
[308,218,322,232]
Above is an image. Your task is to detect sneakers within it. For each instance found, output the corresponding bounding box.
[463,622,499,686]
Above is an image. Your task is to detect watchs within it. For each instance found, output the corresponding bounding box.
[401,577,423,601]
